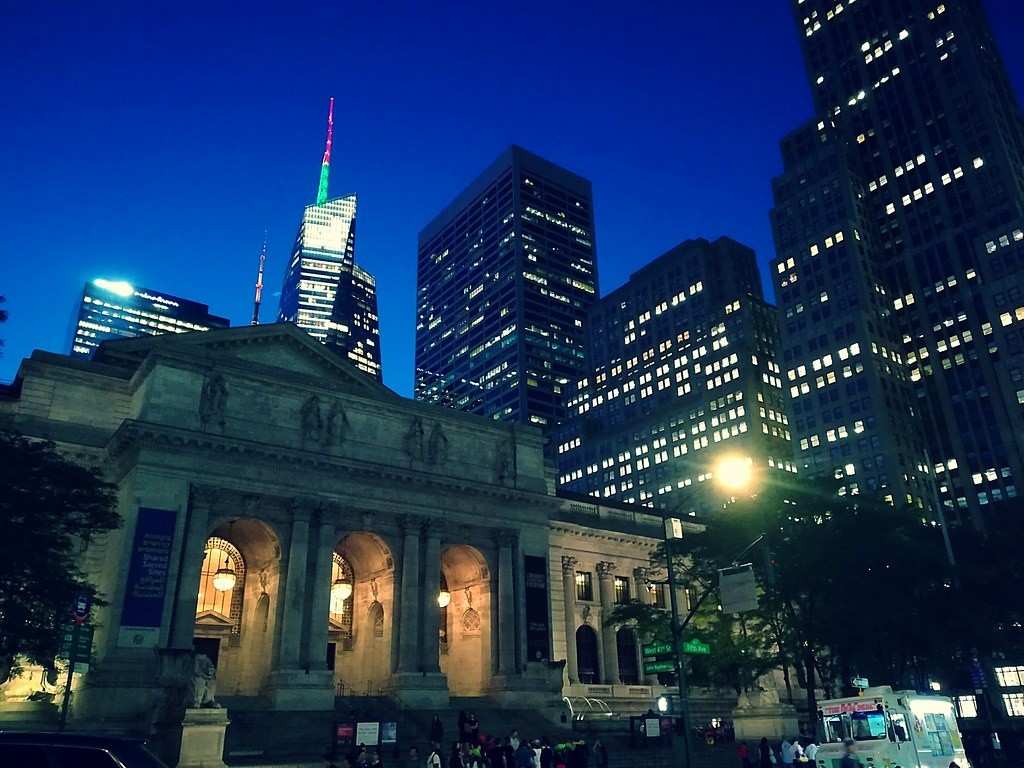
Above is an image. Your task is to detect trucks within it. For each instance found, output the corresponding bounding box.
[812,674,971,768]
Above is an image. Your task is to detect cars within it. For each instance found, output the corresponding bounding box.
[0,731,167,768]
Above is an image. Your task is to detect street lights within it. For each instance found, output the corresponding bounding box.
[660,448,757,768]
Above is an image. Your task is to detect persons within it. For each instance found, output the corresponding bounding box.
[738,737,819,768]
[893,720,906,741]
[800,721,811,738]
[914,715,921,732]
[840,741,864,768]
[354,707,610,768]
[704,717,727,746]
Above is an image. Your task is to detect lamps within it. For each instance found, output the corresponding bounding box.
[439,586,450,608]
[212,556,237,591]
[334,563,352,600]
